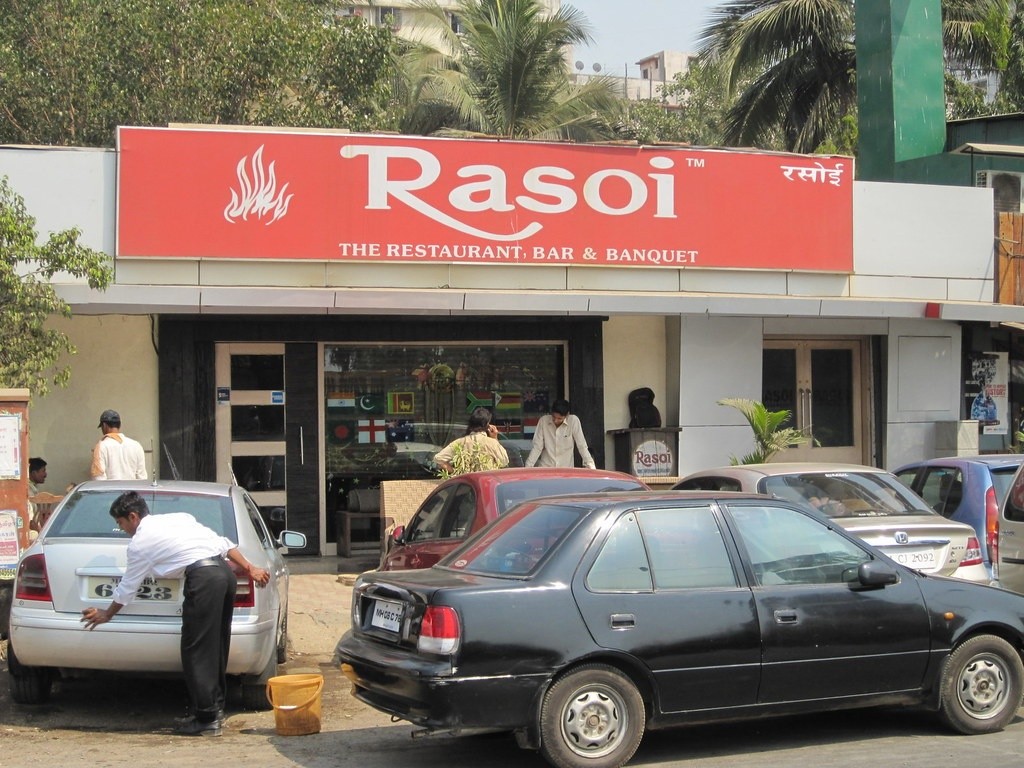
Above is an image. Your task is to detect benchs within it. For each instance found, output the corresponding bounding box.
[336,510,380,559]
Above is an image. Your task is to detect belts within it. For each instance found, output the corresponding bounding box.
[185,560,219,576]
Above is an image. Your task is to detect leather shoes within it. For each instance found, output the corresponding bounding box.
[173,716,222,737]
[174,709,226,728]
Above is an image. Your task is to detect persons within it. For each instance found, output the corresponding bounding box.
[80,492,270,738]
[524,398,597,469]
[27,457,51,545]
[91,410,148,480]
[433,406,510,478]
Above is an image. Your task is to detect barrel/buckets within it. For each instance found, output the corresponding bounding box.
[266,675,324,739]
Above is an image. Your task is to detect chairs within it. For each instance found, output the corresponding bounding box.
[28,493,65,532]
[933,480,962,515]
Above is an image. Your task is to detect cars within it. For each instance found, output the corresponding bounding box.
[664,460,989,586]
[331,486,1024,768]
[370,466,659,573]
[233,421,525,542]
[888,451,1024,597]
[4,478,308,712]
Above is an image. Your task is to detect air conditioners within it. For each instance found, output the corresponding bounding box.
[975,169,1024,212]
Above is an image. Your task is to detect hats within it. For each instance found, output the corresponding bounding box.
[97,410,121,427]
